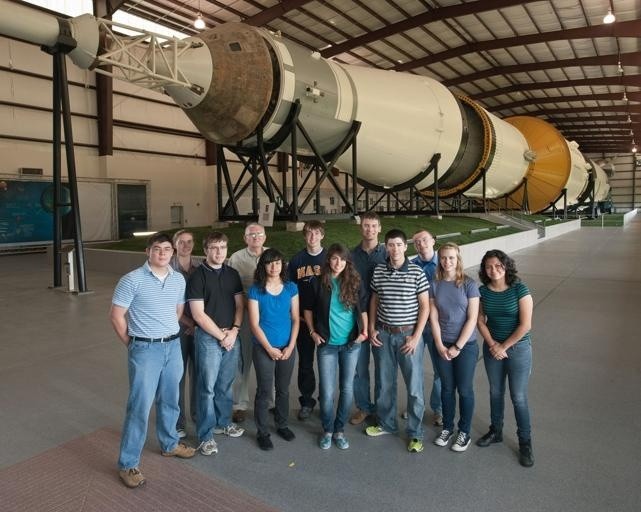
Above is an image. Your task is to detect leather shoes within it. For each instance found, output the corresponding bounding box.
[518,440,536,469]
[477,429,503,447]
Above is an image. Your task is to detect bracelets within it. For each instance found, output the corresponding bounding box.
[361,332,368,339]
[310,328,316,336]
[232,324,240,336]
[454,343,461,351]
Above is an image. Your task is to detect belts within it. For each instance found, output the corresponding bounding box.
[128,331,181,344]
[376,319,416,334]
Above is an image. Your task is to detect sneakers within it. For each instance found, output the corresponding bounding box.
[278,425,296,442]
[177,428,187,441]
[401,411,410,420]
[176,427,187,441]
[255,429,274,451]
[160,441,196,458]
[364,424,394,437]
[332,434,351,450]
[407,437,425,452]
[212,421,247,440]
[434,428,456,447]
[431,413,444,427]
[118,465,148,489]
[450,430,473,453]
[349,407,370,425]
[232,409,246,423]
[319,431,332,450]
[196,437,219,457]
[299,404,313,420]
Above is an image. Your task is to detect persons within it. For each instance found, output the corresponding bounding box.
[110,233,196,488]
[186,231,244,456]
[349,211,390,425]
[227,223,275,422]
[286,219,328,421]
[247,249,299,450]
[169,230,203,440]
[363,228,430,452]
[304,243,368,449]
[402,230,443,426]
[478,250,535,467]
[428,242,481,452]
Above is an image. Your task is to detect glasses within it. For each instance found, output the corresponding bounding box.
[149,245,173,253]
[245,232,267,238]
